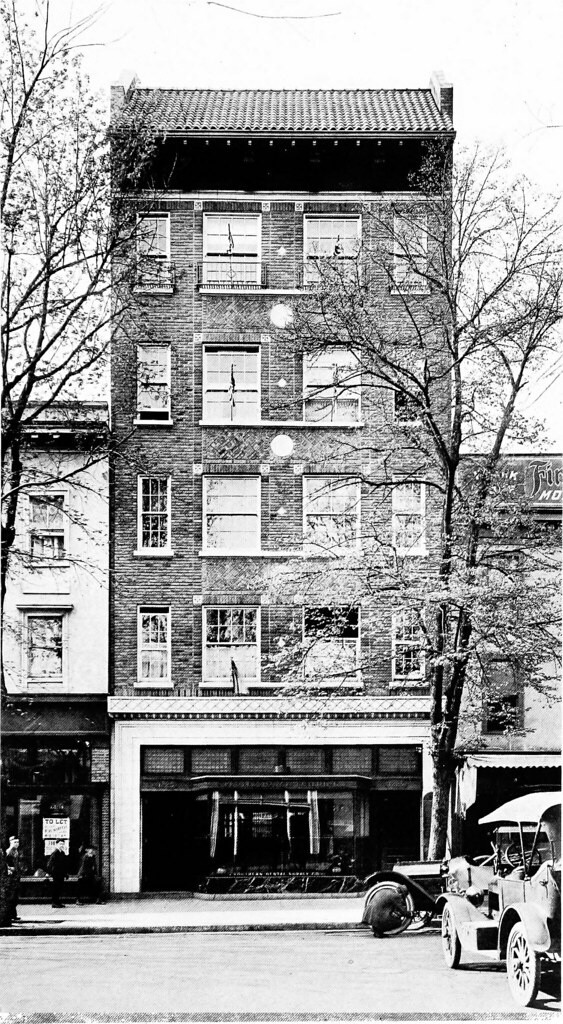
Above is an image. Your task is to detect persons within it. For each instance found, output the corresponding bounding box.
[5,835,24,919]
[46,840,69,908]
[76,844,105,905]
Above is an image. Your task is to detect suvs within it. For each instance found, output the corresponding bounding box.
[431,791,563,1010]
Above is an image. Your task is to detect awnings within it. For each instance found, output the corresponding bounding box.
[457,752,561,817]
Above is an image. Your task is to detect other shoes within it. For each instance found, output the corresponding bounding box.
[14,917,21,921]
[76,900,82,906]
[52,903,65,908]
